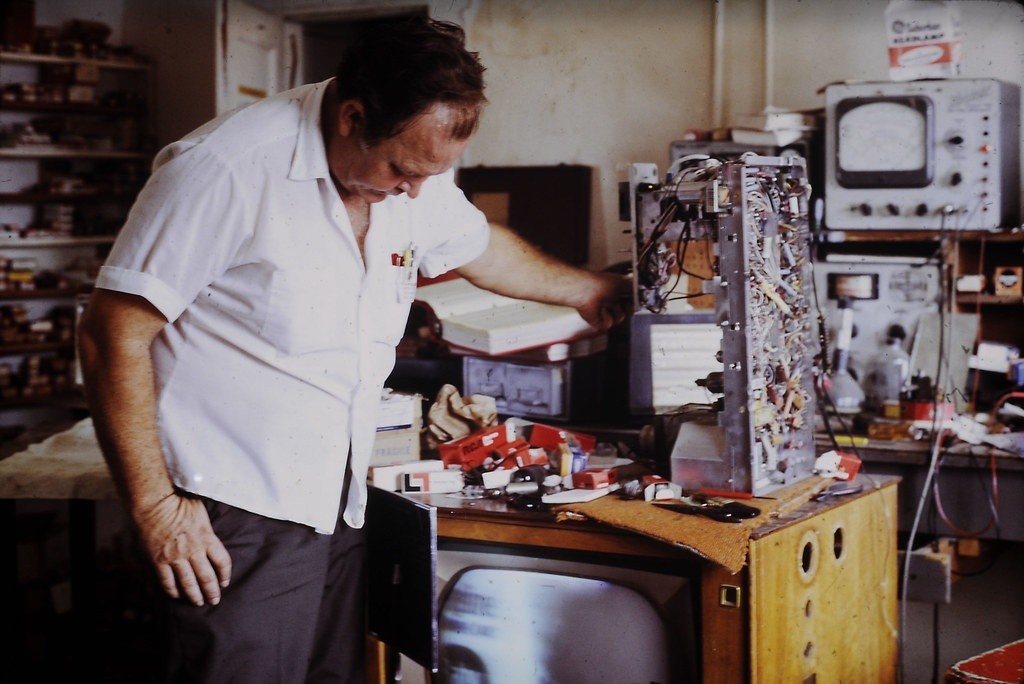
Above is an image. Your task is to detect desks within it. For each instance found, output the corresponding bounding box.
[0,416,901,684]
[812,426,1024,684]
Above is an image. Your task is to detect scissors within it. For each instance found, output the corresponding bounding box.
[653,501,762,523]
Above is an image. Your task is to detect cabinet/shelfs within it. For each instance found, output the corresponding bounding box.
[0,0,155,458]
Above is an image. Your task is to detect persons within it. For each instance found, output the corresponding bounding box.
[77,20,647,683]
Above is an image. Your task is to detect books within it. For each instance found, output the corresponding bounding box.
[415,278,599,357]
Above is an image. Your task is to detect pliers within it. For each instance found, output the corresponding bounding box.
[810,481,864,502]
